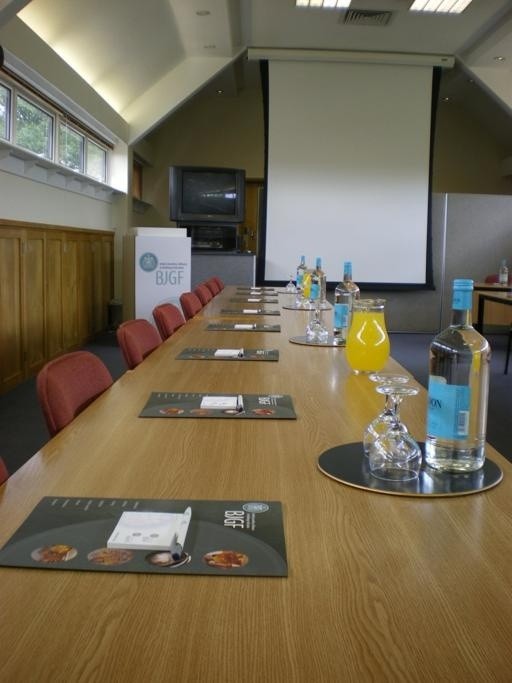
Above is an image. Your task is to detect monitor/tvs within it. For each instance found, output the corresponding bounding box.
[168,165,246,223]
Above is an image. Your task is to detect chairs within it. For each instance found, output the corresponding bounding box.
[116,318,164,370]
[486,275,512,284]
[153,303,187,342]
[0,457,10,485]
[180,275,225,320]
[36,351,114,438]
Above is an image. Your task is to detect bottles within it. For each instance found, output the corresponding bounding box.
[498,258,508,285]
[310,257,327,304]
[424,277,493,472]
[296,254,307,286]
[345,298,391,374]
[334,261,360,346]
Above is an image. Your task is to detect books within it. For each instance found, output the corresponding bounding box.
[138,391,297,419]
[205,287,280,331]
[175,347,280,361]
[0,497,288,577]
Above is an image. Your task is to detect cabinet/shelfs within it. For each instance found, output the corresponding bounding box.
[176,221,241,255]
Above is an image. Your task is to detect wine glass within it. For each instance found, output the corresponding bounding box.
[296,288,311,308]
[286,272,296,291]
[363,371,424,481]
[304,300,329,343]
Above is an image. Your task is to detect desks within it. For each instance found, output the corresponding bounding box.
[473,283,512,291]
[477,290,512,375]
[0,282,512,682]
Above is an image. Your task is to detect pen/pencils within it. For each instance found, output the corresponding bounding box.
[253,323,256,329]
[170,506,192,561]
[239,347,244,357]
[237,394,243,412]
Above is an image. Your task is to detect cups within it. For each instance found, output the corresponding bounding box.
[302,268,315,297]
